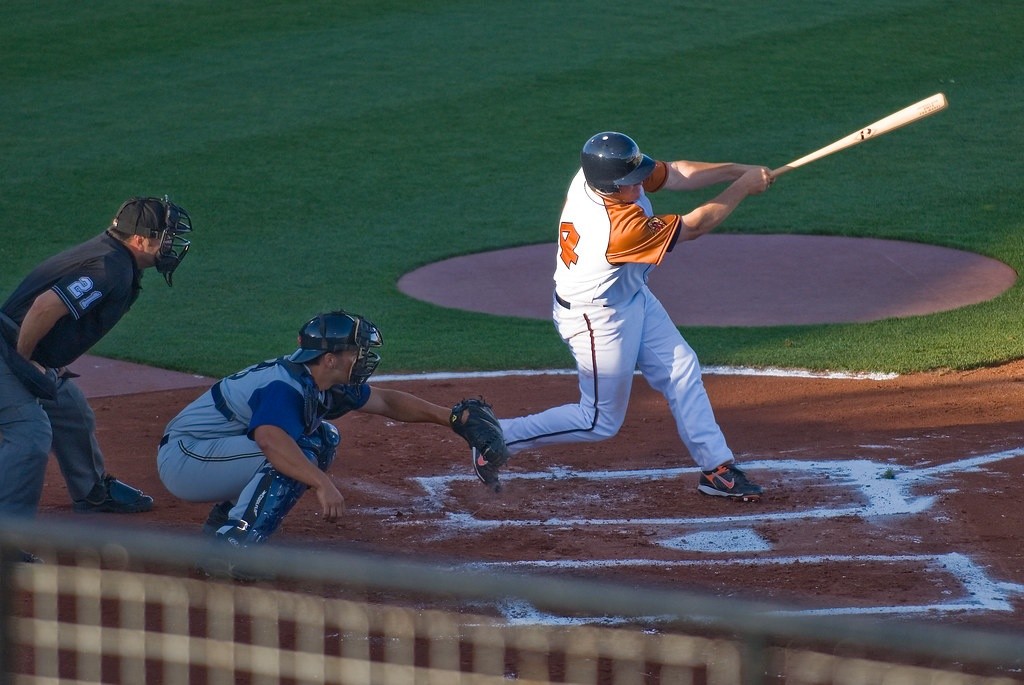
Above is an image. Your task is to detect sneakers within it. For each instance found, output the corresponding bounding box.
[697,462,763,502]
[12,549,45,565]
[73,479,154,513]
[473,447,500,492]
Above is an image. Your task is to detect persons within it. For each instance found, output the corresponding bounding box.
[472,130,775,502]
[156,310,506,585]
[0,193,194,563]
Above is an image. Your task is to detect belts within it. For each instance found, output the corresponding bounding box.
[556,294,609,309]
[160,434,169,449]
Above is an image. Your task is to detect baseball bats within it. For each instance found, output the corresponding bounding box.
[769,90,950,178]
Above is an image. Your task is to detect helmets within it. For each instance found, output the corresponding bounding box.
[582,132,655,193]
[287,312,356,363]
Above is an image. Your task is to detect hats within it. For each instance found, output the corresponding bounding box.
[114,197,187,239]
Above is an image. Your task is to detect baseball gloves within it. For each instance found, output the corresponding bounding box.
[449,393,511,486]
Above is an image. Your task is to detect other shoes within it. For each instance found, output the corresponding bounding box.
[205,503,232,534]
